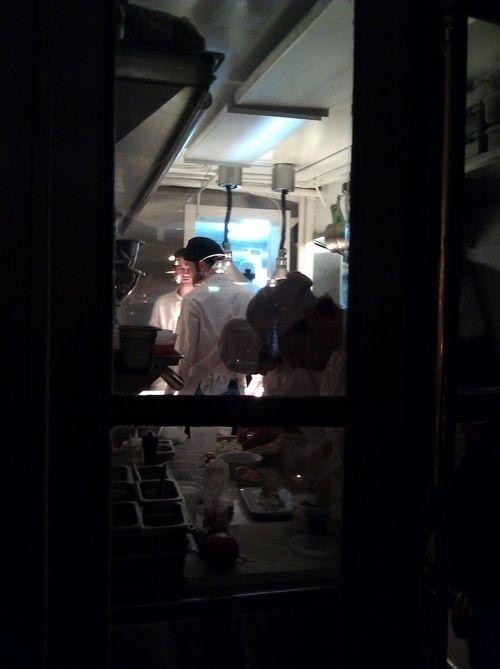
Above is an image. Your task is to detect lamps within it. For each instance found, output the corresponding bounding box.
[272,163,295,280]
[193,166,251,285]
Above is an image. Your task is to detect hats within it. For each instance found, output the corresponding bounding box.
[246,270,318,345]
[217,317,265,375]
[184,237,225,262]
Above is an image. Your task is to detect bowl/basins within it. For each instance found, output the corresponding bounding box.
[221,451,263,473]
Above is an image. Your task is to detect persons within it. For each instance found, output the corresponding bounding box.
[148,247,200,397]
[162,236,262,401]
[220,277,345,455]
